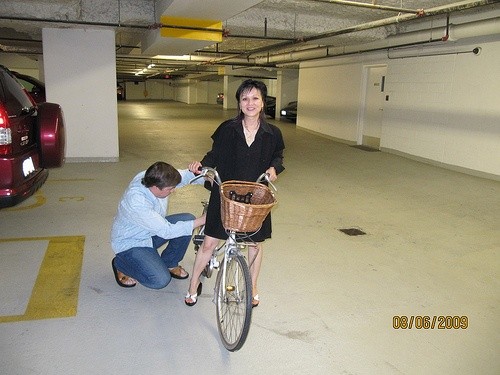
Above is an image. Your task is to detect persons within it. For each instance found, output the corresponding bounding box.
[184,79,285,306]
[112,161,214,291]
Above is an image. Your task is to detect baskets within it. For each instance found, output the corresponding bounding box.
[217,180,278,231]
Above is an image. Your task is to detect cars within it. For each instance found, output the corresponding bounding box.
[216,92,298,119]
[117,84,124,100]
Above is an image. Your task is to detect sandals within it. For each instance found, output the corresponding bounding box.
[112,257,138,288]
[170,265,190,280]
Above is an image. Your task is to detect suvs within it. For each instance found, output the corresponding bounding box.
[0,64,67,210]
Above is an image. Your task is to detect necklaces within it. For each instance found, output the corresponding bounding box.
[243,119,260,136]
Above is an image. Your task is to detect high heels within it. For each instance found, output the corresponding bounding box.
[185,281,203,307]
[249,293,260,307]
